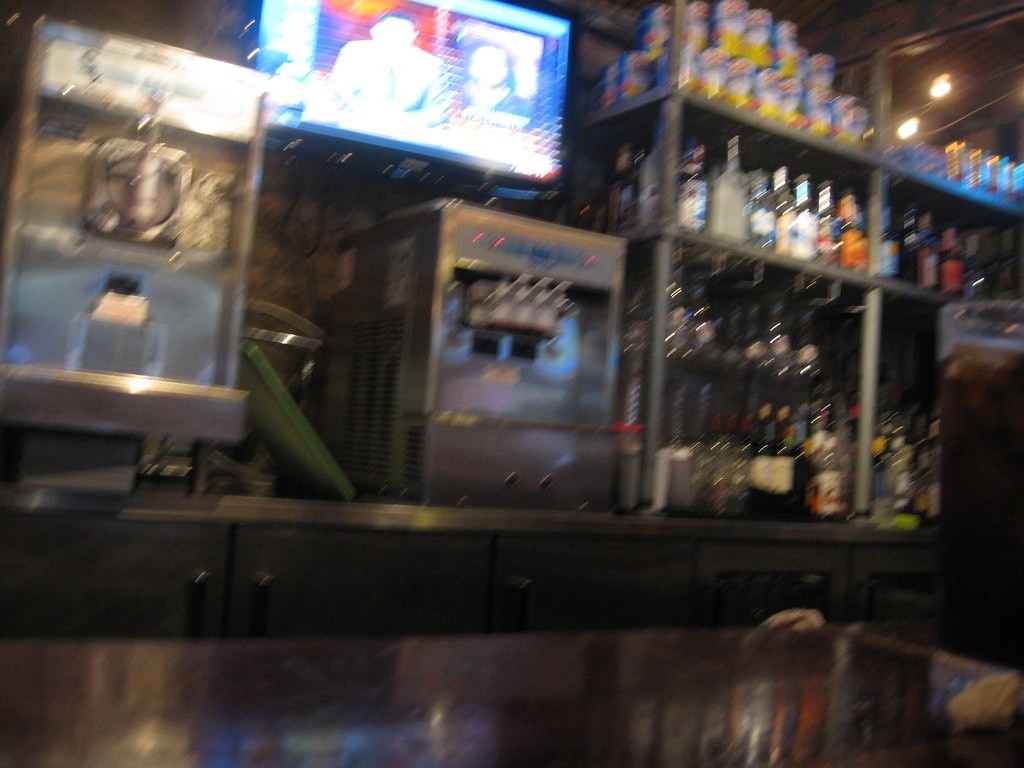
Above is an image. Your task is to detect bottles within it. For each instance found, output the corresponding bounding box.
[665,279,820,379]
[878,204,1016,301]
[685,377,940,521]
[607,135,869,276]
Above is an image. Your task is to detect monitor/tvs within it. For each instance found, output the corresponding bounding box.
[239,1,584,220]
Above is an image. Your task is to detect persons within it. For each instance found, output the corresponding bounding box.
[324,10,446,122]
[456,38,537,126]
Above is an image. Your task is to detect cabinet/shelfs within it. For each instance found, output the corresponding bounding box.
[571,0,1024,520]
